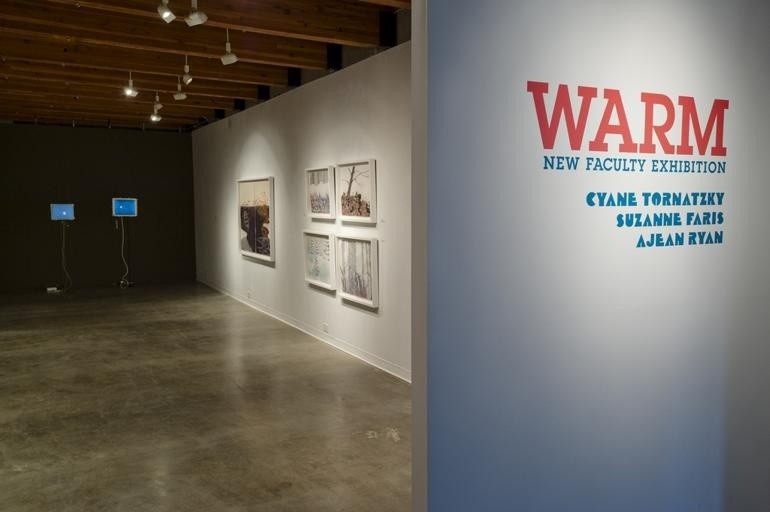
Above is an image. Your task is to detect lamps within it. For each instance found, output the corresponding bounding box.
[120,1,242,124]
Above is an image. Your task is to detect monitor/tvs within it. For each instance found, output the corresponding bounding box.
[50,203,75,221]
[111,197,139,217]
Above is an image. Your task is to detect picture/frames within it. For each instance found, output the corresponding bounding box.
[303,164,336,221]
[300,229,338,294]
[333,232,380,311]
[235,175,277,266]
[335,156,378,226]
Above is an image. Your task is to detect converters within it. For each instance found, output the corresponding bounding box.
[46,286,57,294]
[120,280,137,286]
